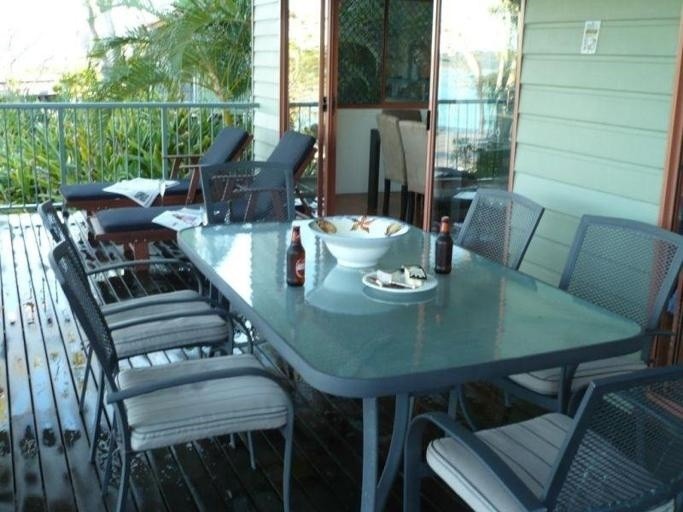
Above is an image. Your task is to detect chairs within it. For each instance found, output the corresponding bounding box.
[398,120,427,226]
[59,126,252,215]
[87,130,315,268]
[447,214,683,463]
[48,240,293,512]
[37,199,230,462]
[454,189,546,270]
[377,114,407,221]
[403,363,683,512]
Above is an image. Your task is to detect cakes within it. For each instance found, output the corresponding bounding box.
[403,264,427,287]
[377,267,400,284]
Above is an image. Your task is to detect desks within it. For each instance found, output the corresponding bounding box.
[178,215,646,512]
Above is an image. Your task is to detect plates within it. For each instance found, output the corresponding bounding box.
[363,268,439,292]
[363,291,437,308]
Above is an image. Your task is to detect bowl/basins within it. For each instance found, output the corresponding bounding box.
[307,215,409,264]
[303,263,400,316]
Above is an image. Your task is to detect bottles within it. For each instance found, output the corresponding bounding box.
[285,225,306,286]
[435,278,448,326]
[285,287,301,348]
[434,216,452,275]
